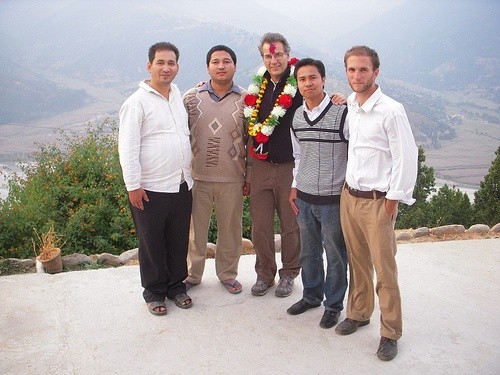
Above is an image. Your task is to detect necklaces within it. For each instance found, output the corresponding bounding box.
[244,58,299,160]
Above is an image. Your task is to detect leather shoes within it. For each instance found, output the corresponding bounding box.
[319,310,340,329]
[287,298,321,315]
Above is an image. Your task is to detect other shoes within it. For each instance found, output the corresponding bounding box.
[147,301,167,315]
[171,294,192,309]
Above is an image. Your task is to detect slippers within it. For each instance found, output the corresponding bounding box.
[182,279,197,291]
[222,281,242,294]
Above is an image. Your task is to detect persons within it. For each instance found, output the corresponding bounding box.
[197,32,347,297]
[181,45,250,294]
[117,41,195,316]
[335,46,418,361]
[287,58,348,329]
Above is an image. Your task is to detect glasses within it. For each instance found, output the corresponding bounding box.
[261,52,284,61]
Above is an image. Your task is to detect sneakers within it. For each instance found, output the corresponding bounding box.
[275,275,294,297]
[335,318,370,335]
[377,336,397,361]
[251,280,274,295]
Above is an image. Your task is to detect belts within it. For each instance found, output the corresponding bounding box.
[345,183,386,199]
